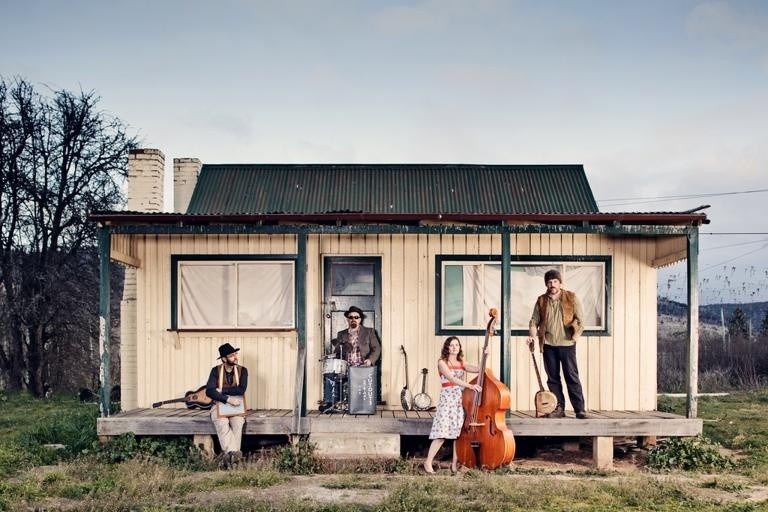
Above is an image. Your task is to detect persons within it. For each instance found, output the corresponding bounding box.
[319,307,381,414]
[423,337,490,475]
[206,343,248,469]
[527,270,587,419]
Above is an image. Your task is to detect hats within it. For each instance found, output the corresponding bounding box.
[344,306,365,318]
[217,343,239,359]
[545,270,561,285]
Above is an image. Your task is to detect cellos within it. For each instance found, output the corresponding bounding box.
[455,309,515,470]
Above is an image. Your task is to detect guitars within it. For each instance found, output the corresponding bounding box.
[153,385,214,410]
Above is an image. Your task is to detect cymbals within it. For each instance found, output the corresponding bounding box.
[331,339,353,353]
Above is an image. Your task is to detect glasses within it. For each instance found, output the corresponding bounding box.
[347,316,359,319]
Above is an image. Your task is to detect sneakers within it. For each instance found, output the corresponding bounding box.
[219,451,242,470]
[319,404,336,413]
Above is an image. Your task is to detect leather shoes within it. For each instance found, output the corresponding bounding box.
[424,465,436,475]
[547,410,565,418]
[576,411,588,418]
[450,465,457,473]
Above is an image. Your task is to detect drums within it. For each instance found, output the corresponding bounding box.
[321,359,347,379]
